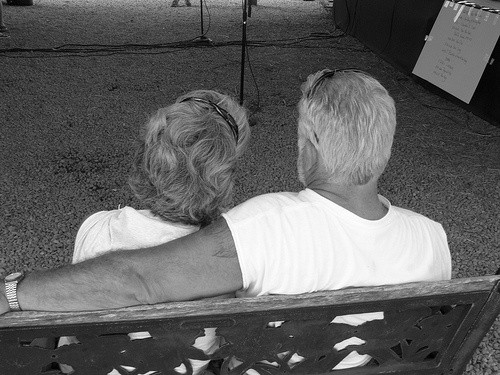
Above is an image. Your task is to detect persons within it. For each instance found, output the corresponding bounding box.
[50,88,251,375]
[0,66,455,375]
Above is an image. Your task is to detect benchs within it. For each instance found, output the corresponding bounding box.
[0,266,499,375]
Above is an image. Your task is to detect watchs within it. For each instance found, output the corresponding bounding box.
[3,270,27,312]
